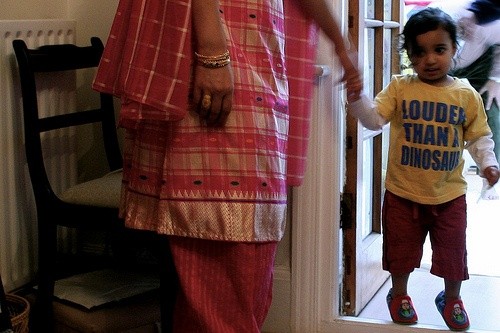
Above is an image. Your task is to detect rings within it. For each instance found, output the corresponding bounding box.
[202,94,211,109]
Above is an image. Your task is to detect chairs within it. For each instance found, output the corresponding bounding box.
[13,36,180,333]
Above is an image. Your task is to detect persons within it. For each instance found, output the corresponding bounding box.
[457,0,500,40]
[403,0,500,200]
[91,0,364,333]
[332,6,500,331]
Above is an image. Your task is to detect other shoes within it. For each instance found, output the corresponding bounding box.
[435,290,470,332]
[387,288,417,325]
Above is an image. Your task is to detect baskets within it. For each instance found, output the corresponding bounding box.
[4,293,30,333]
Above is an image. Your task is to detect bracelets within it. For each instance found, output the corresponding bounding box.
[192,49,231,69]
[488,76,500,82]
